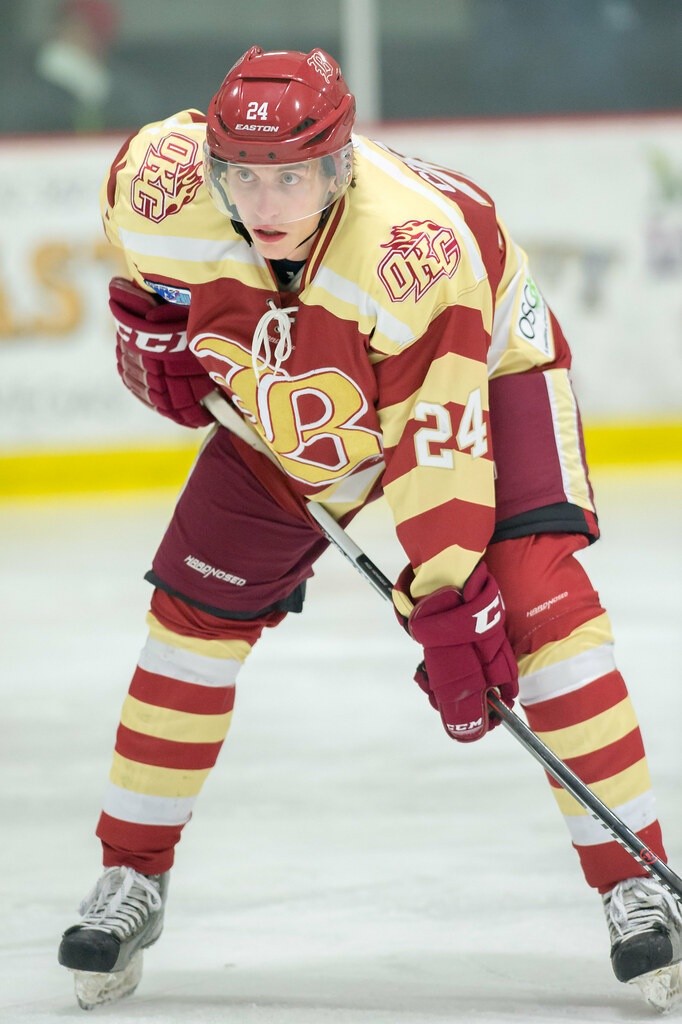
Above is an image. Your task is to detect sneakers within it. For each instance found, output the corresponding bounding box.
[56,867,174,1010]
[598,869,682,1016]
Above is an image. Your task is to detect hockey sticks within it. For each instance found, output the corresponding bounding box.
[203,389,682,895]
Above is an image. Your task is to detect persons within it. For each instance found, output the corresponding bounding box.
[55,45,682,1015]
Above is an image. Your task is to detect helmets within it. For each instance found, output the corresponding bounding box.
[208,44,356,185]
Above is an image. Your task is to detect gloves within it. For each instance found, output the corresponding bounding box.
[405,561,520,742]
[110,272,221,431]
